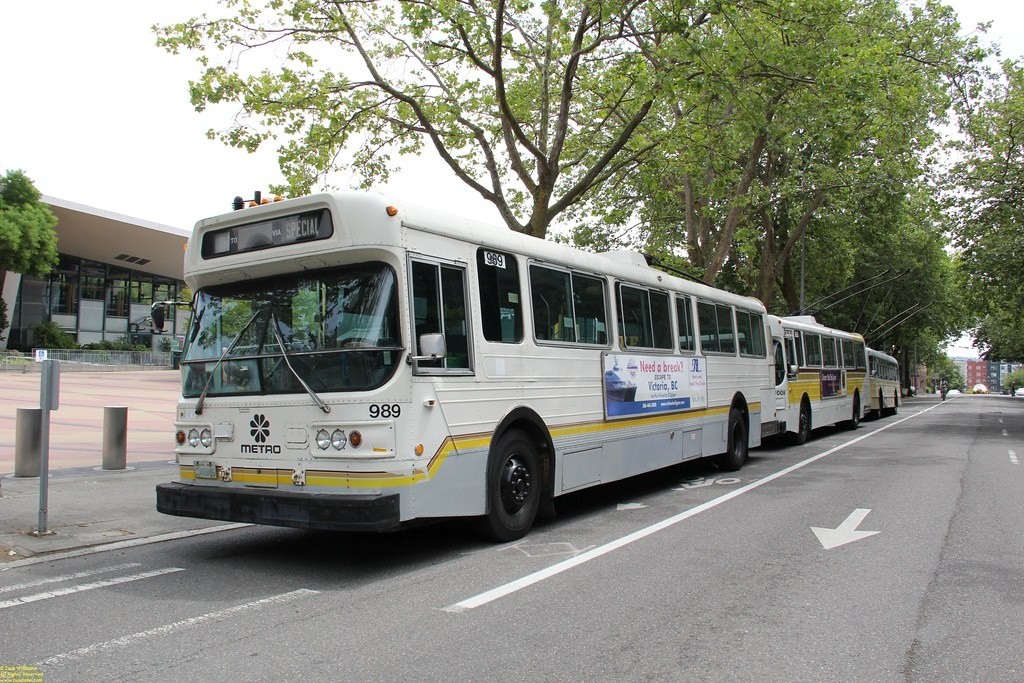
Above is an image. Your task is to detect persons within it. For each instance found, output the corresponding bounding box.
[940,377,949,398]
[1011,382,1016,396]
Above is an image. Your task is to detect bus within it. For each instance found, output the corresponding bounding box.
[866,347,902,420]
[768,315,871,445]
[151,192,778,542]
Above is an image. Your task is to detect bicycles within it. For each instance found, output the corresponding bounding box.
[941,386,948,401]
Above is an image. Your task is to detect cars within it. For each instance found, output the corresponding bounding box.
[972,383,986,394]
